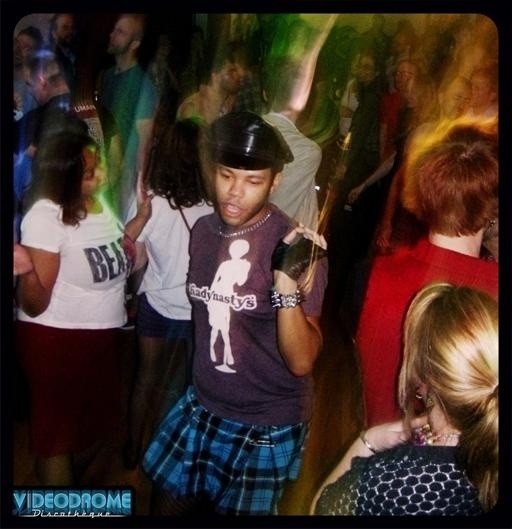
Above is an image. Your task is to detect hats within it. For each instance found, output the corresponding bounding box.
[207,111,294,176]
